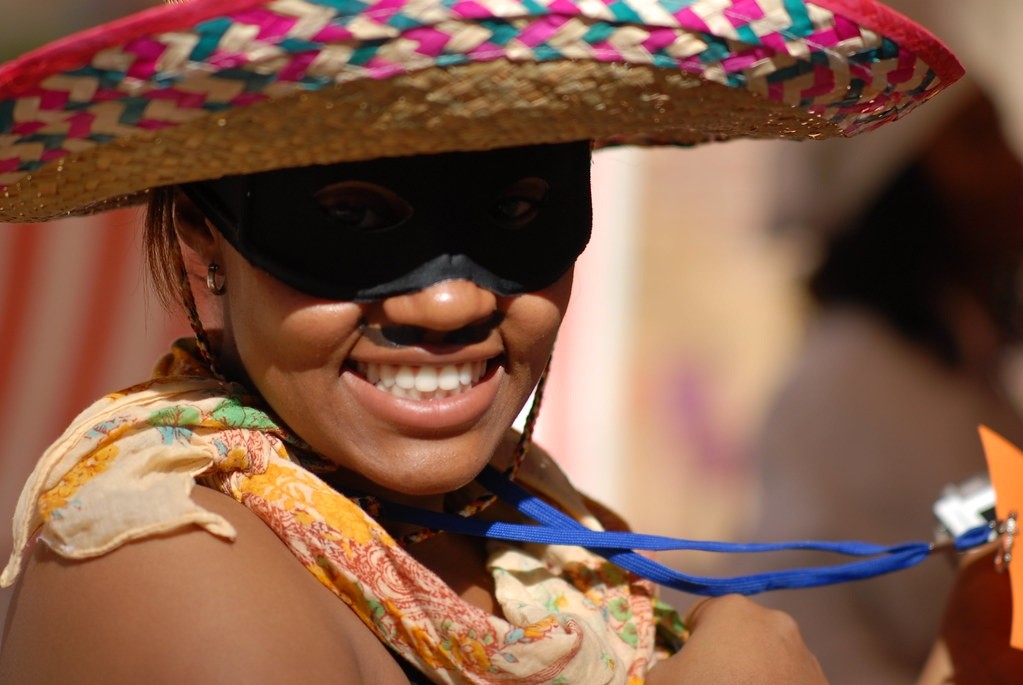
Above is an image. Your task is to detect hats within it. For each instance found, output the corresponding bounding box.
[1,0,964,227]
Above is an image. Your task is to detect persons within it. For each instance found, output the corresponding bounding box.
[656,49,1023,682]
[0,0,1023,685]
